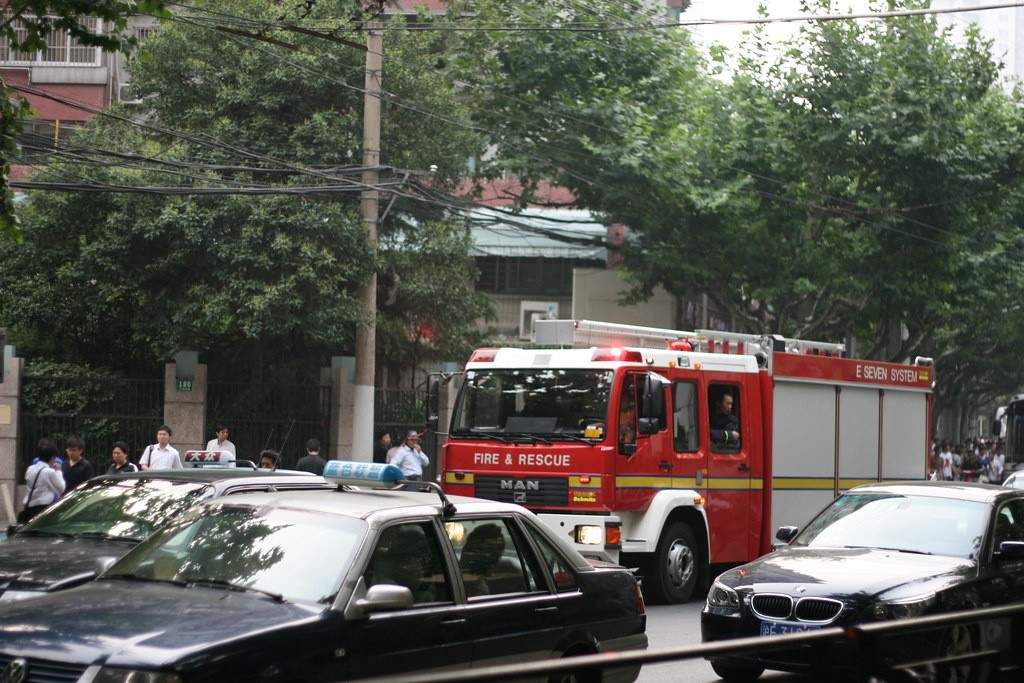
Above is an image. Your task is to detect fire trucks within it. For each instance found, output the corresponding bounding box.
[413,315,945,604]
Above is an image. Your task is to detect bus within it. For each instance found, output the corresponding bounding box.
[991,393,1024,482]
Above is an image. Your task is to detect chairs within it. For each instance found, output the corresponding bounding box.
[374,548,424,594]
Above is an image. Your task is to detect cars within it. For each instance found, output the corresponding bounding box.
[0,461,651,683]
[0,463,363,610]
[699,481,1024,683]
[997,469,1024,521]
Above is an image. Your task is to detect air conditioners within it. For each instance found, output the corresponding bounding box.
[118,83,144,105]
[519,301,560,340]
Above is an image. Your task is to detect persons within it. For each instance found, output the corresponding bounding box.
[106,442,138,473]
[586,386,641,444]
[929,436,1007,482]
[373,429,429,481]
[18,436,94,528]
[296,439,328,476]
[711,394,739,450]
[260,450,281,470]
[139,426,184,471]
[203,425,237,469]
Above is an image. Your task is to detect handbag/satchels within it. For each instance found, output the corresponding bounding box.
[17,511,26,524]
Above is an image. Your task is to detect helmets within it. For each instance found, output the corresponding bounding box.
[406,431,419,441]
